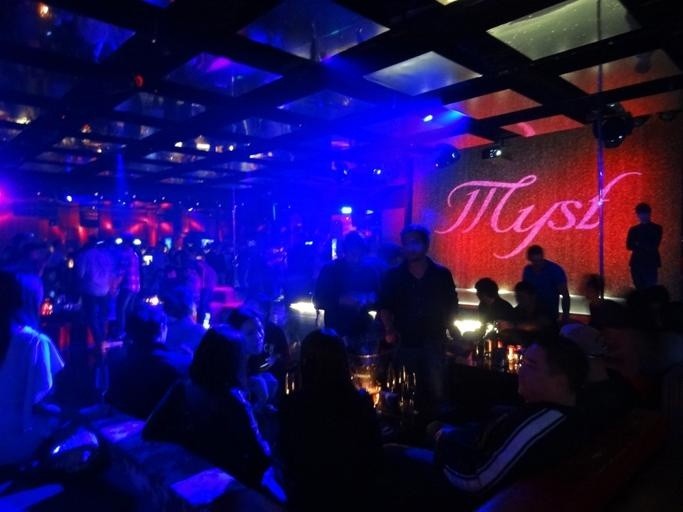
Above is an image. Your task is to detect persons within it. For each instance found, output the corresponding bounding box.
[1,215,682,510]
[621,202,663,287]
